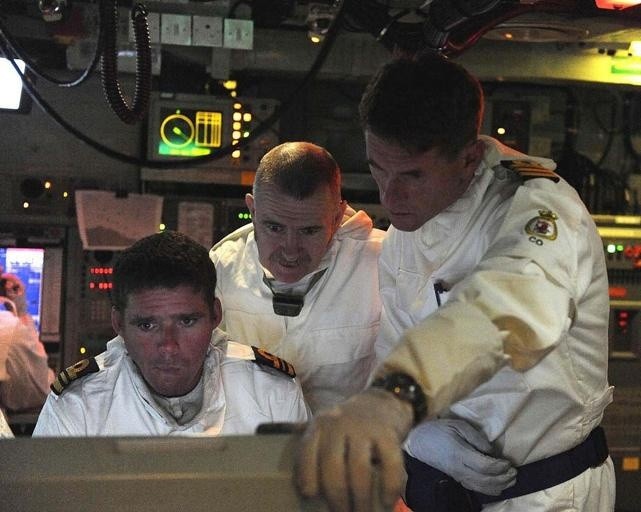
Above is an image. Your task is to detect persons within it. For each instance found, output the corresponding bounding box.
[207,141,389,420]
[251,50,619,512]
[30,227,315,438]
[0,261,59,441]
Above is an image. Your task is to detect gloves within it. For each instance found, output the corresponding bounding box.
[298,389,517,512]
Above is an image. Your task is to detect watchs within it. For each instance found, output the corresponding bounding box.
[370,371,429,426]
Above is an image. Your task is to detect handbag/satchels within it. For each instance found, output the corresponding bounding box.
[406,464,481,512]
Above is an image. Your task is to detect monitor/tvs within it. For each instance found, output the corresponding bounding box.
[0,247,45,336]
[0,428,383,512]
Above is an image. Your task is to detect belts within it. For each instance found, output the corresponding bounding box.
[404,426,609,504]
